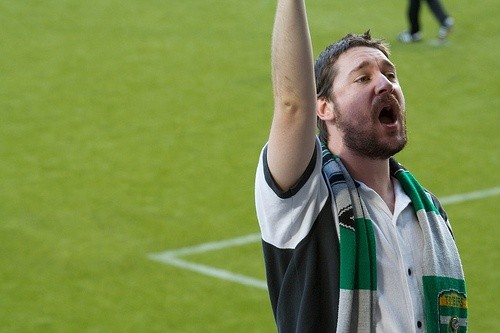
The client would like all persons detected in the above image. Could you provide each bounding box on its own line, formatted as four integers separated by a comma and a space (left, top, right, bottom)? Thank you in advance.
398, 0, 457, 43
255, 1, 468, 333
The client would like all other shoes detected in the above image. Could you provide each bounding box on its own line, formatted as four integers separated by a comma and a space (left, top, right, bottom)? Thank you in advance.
437, 16, 454, 39
398, 32, 421, 42
